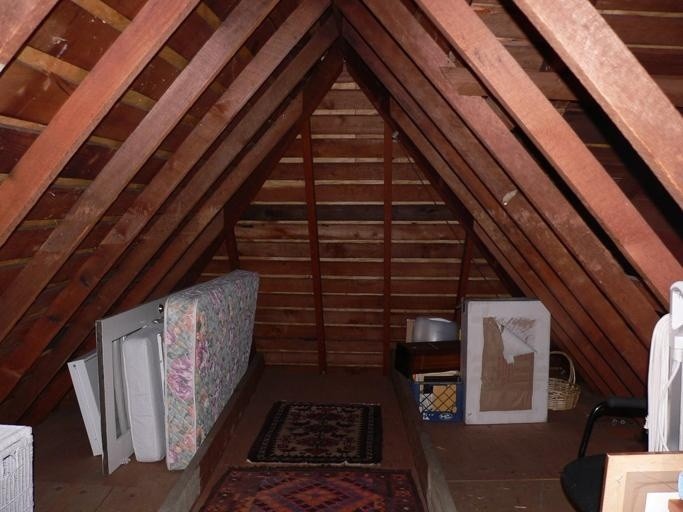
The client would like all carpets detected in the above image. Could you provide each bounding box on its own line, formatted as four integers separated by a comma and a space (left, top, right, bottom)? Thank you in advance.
196, 399, 428, 511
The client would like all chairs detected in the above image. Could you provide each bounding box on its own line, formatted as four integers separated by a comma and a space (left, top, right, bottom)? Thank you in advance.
562, 397, 647, 511
